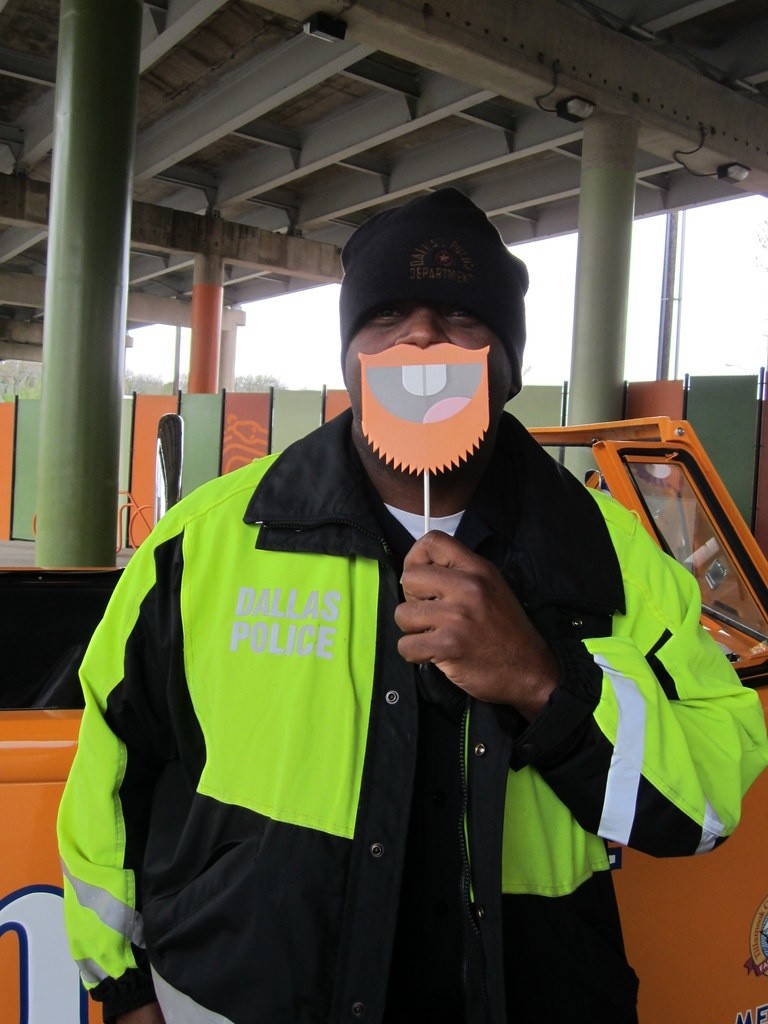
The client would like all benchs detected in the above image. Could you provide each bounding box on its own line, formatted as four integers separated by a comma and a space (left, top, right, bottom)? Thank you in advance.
0, 567, 125, 710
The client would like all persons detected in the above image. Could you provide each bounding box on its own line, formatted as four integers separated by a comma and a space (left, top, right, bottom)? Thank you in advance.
58, 186, 768, 1023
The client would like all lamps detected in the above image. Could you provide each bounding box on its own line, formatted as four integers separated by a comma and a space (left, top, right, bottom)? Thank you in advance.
717, 162, 752, 184
300, 10, 349, 43
556, 94, 597, 124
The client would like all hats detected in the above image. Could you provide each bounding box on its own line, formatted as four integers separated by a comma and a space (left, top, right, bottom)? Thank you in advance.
339, 186, 529, 392
